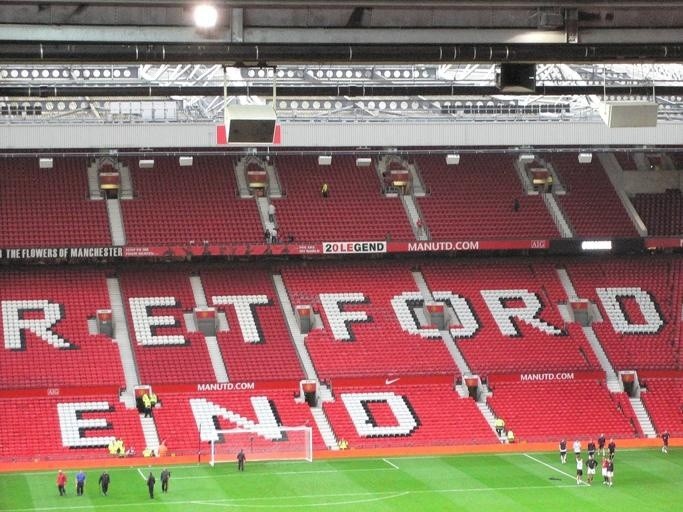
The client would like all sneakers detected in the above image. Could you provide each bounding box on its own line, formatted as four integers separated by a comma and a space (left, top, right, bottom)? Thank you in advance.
588, 479, 594, 487
561, 460, 567, 464
597, 452, 606, 457
602, 481, 613, 488
577, 479, 582, 485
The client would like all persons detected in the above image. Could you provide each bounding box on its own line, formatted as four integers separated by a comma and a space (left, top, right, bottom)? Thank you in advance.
76, 470, 85, 496
383, 230, 392, 242
504, 426, 514, 443
546, 174, 553, 192
149, 391, 158, 406
415, 218, 423, 236
107, 438, 156, 458
511, 193, 520, 212
160, 467, 170, 492
271, 228, 277, 244
558, 433, 616, 488
321, 182, 328, 196
98, 471, 110, 496
660, 430, 670, 454
56, 470, 66, 496
144, 398, 151, 418
268, 202, 275, 222
265, 228, 270, 243
147, 472, 155, 499
337, 437, 348, 450
141, 393, 148, 412
236, 449, 245, 471
493, 416, 504, 437
287, 232, 293, 242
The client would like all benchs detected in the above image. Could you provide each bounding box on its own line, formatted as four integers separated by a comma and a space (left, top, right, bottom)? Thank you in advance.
0, 150, 683, 464
